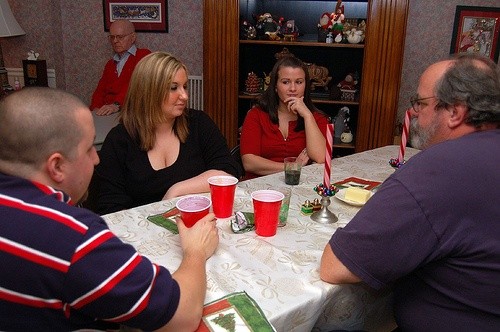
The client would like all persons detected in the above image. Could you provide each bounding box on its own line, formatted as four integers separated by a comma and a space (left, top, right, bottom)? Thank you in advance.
0, 86, 219, 332
317, 12, 333, 31
336, 73, 359, 90
82, 50, 242, 215
319, 51, 500, 332
237, 56, 330, 180
89, 18, 152, 117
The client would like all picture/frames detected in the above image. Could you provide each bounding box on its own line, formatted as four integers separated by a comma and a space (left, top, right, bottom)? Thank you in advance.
102, 0, 169, 34
449, 5, 500, 66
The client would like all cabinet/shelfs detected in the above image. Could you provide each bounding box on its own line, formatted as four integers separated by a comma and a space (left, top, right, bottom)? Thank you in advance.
203, 0, 410, 180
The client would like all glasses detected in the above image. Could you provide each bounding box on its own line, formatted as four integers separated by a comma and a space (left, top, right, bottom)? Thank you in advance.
107, 32, 132, 41
410, 96, 441, 113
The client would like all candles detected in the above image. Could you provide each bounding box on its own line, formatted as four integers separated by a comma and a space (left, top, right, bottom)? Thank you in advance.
393, 107, 411, 167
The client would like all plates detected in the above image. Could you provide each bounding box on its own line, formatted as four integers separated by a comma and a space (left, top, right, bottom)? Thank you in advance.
334, 188, 375, 206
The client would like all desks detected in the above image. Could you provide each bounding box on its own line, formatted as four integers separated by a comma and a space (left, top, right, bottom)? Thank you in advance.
100, 145, 420, 332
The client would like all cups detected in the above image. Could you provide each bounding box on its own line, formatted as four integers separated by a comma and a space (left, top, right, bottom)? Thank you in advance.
266, 186, 291, 227
284, 157, 303, 185
251, 190, 285, 237
208, 175, 239, 219
175, 195, 212, 228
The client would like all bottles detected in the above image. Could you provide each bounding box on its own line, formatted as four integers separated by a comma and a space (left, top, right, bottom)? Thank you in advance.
14, 76, 19, 91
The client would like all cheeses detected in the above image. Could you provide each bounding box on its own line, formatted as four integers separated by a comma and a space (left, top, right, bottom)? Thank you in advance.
345, 186, 370, 203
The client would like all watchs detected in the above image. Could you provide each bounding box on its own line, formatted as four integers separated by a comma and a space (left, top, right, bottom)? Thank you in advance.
113, 102, 121, 111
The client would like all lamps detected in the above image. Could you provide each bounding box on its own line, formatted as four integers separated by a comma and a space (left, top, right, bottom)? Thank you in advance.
0, 0, 27, 89
